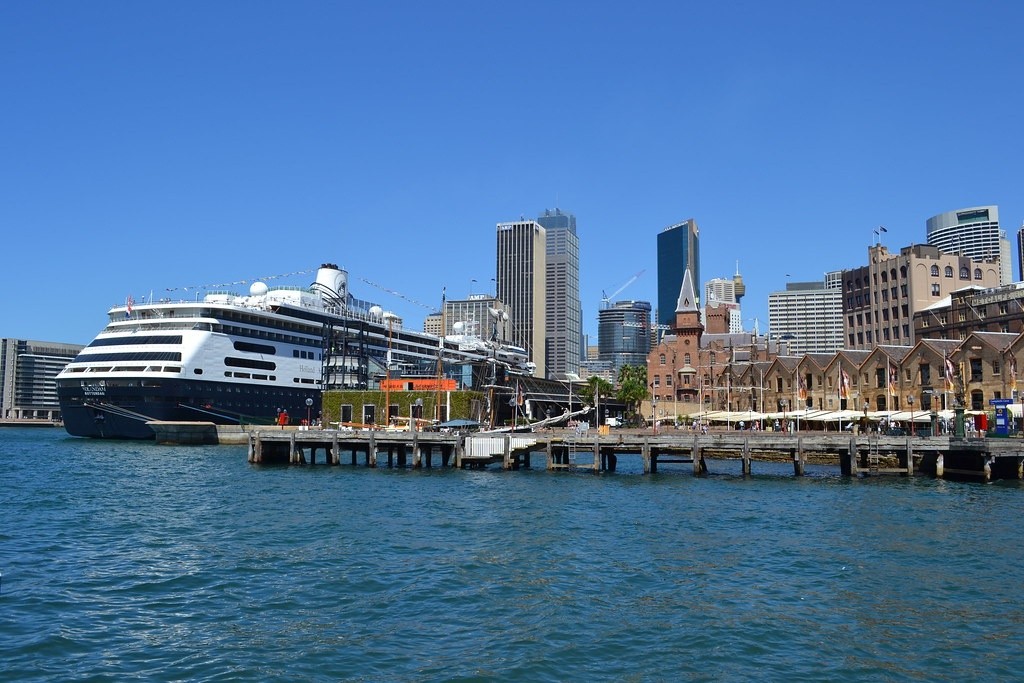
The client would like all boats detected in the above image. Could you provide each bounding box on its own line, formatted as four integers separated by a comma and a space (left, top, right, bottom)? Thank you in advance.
54, 263, 537, 440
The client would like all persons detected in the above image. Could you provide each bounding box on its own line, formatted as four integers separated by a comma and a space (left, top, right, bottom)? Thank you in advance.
880, 418, 885, 429
568, 419, 584, 430
751, 421, 761, 431
675, 421, 679, 428
692, 419, 697, 429
775, 419, 779, 431
890, 419, 901, 430
739, 420, 745, 430
702, 424, 709, 434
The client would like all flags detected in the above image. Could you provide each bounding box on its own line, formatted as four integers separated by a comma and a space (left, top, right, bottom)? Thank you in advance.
840, 368, 850, 399
874, 230, 879, 235
798, 373, 807, 399
889, 366, 896, 396
126, 299, 131, 317
880, 227, 887, 232
1010, 350, 1017, 390
944, 359, 955, 390
518, 384, 523, 406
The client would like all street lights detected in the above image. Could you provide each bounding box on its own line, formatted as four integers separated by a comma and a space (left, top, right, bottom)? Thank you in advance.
951, 398, 957, 430
508, 399, 516, 434
704, 409, 708, 425
385, 314, 397, 426
748, 407, 752, 432
415, 398, 423, 432
546, 409, 551, 430
932, 389, 940, 437
908, 395, 915, 438
650, 400, 657, 436
780, 398, 788, 435
804, 406, 809, 432
665, 409, 669, 430
863, 402, 869, 429
306, 398, 313, 430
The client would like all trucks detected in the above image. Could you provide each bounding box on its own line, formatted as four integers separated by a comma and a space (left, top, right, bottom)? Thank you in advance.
604, 417, 624, 427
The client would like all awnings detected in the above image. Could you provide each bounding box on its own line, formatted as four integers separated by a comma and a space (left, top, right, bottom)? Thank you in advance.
643, 410, 989, 424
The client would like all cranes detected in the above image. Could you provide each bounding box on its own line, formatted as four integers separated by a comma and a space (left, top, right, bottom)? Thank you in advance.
601, 269, 645, 309
